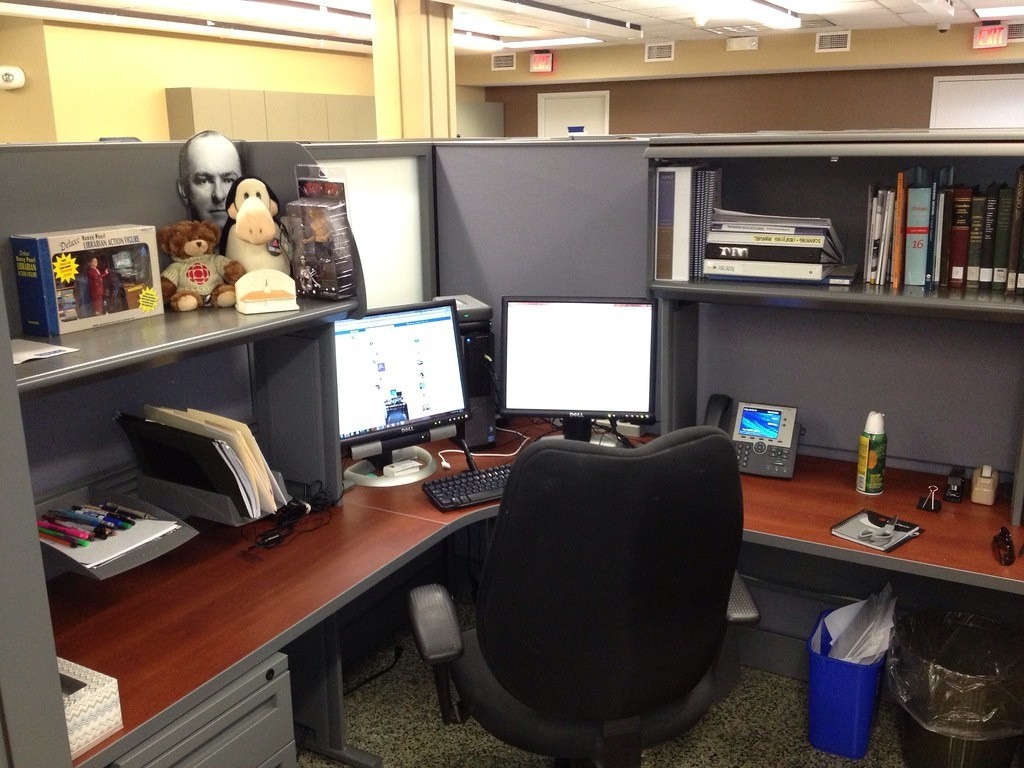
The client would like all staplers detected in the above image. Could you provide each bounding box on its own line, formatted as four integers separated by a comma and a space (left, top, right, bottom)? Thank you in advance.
943, 464, 967, 503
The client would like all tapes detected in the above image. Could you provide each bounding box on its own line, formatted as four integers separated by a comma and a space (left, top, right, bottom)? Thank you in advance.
982, 465, 993, 478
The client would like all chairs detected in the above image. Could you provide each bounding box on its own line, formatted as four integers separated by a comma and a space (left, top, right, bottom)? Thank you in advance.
408, 429, 759, 768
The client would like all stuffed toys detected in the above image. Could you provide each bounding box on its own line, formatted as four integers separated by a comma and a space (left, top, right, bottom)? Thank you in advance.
157, 175, 292, 313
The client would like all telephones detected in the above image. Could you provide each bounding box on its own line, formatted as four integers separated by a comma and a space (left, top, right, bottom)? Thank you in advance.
703, 392, 807, 480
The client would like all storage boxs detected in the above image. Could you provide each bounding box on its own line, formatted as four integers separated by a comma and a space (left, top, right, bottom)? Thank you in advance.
8, 222, 165, 340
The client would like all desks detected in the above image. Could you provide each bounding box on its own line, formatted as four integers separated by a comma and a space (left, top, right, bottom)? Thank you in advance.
341, 417, 1024, 599
40, 491, 446, 768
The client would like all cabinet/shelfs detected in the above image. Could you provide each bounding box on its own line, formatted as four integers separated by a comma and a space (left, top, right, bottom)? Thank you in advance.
108, 651, 298, 768
342, 143, 1024, 679
0, 138, 448, 768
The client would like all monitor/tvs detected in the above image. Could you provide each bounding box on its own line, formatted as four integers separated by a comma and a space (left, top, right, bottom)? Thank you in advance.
334, 298, 471, 488
499, 297, 659, 450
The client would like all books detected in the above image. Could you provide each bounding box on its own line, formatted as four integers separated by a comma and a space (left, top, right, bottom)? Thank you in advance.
830, 508, 920, 551
703, 164, 1024, 300
39, 508, 178, 568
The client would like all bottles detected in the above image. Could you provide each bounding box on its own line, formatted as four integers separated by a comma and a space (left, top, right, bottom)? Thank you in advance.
855, 410, 888, 495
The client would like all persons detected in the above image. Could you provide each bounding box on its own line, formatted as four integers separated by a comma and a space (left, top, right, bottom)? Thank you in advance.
88, 257, 109, 315
302, 208, 339, 291
177, 130, 243, 235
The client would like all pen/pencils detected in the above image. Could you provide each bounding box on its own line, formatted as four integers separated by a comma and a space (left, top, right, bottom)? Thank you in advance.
37, 502, 159, 549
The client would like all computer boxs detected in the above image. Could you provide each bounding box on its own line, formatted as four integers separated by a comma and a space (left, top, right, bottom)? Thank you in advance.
448, 330, 496, 451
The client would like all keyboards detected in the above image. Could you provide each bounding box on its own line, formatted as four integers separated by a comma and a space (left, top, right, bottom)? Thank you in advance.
422, 464, 514, 513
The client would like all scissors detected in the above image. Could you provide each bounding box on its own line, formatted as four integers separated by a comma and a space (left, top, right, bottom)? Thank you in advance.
857, 513, 900, 542
